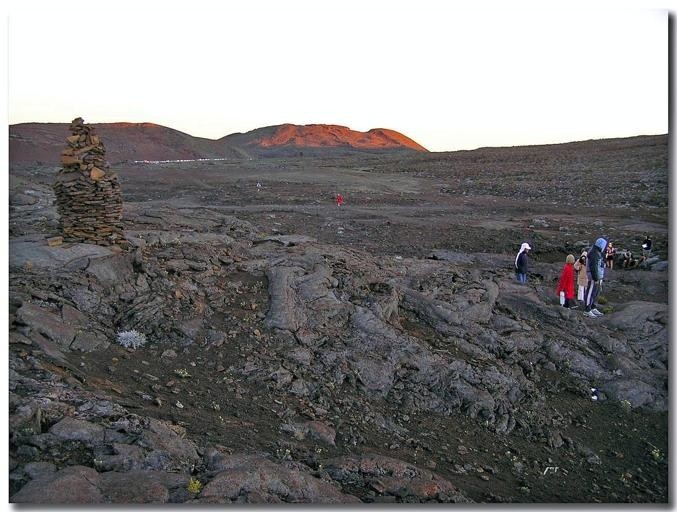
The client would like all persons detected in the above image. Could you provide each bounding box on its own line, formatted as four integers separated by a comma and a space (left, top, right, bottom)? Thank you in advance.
515, 242, 531, 283
556, 254, 575, 307
573, 236, 653, 318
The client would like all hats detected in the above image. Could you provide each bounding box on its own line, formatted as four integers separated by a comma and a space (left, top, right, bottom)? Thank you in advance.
521, 243, 531, 250
608, 243, 612, 248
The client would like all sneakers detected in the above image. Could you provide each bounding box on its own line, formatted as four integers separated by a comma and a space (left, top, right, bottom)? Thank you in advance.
581, 308, 604, 319
